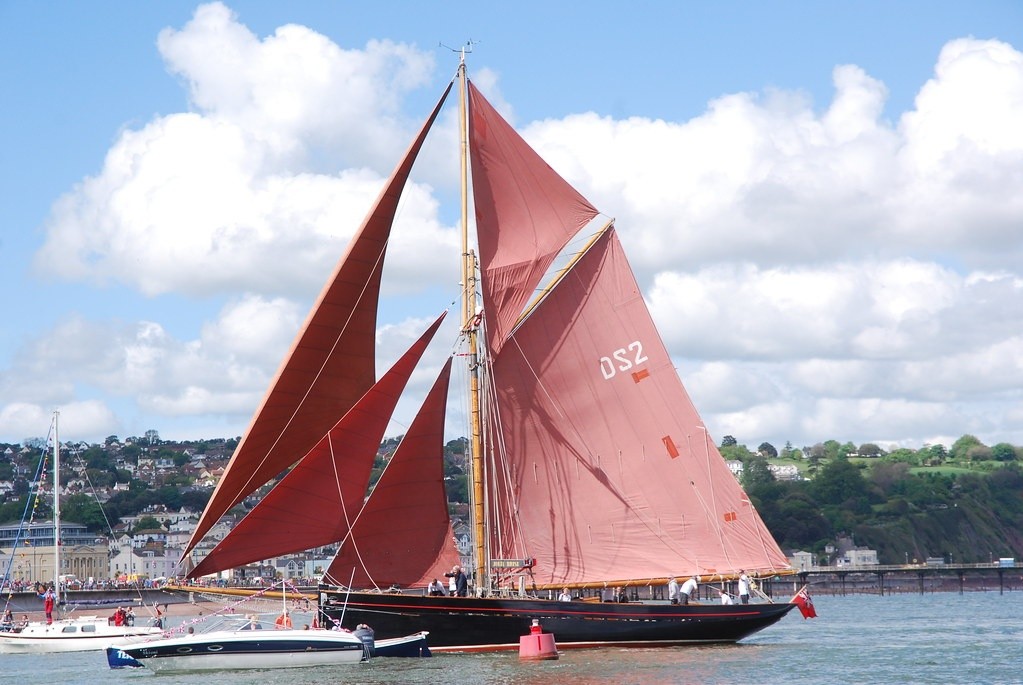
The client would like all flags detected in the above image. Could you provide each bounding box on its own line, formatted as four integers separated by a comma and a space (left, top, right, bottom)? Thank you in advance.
793, 586, 819, 620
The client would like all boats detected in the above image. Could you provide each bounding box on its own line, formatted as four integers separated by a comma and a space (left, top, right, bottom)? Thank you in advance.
106, 578, 373, 673
354, 630, 434, 660
60, 598, 145, 611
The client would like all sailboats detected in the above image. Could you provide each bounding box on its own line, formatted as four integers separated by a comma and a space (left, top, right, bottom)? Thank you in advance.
0, 410, 166, 655
175, 43, 807, 658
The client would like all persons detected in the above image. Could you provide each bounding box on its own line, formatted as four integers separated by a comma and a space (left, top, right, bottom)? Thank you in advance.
719, 589, 733, 605
679, 576, 698, 605
738, 569, 749, 604
37, 587, 57, 625
558, 588, 571, 602
443, 565, 467, 597
0, 609, 30, 633
241, 614, 262, 630
184, 627, 195, 638
5, 575, 321, 593
667, 574, 680, 605
271, 609, 291, 630
108, 606, 137, 626
428, 578, 446, 597
613, 586, 629, 603
